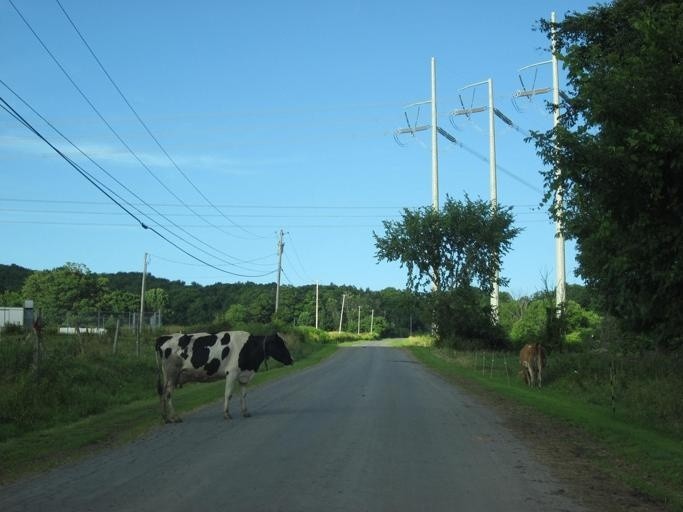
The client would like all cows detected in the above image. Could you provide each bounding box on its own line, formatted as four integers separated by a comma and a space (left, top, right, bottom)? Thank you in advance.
154, 330, 294, 423
519, 342, 549, 390
